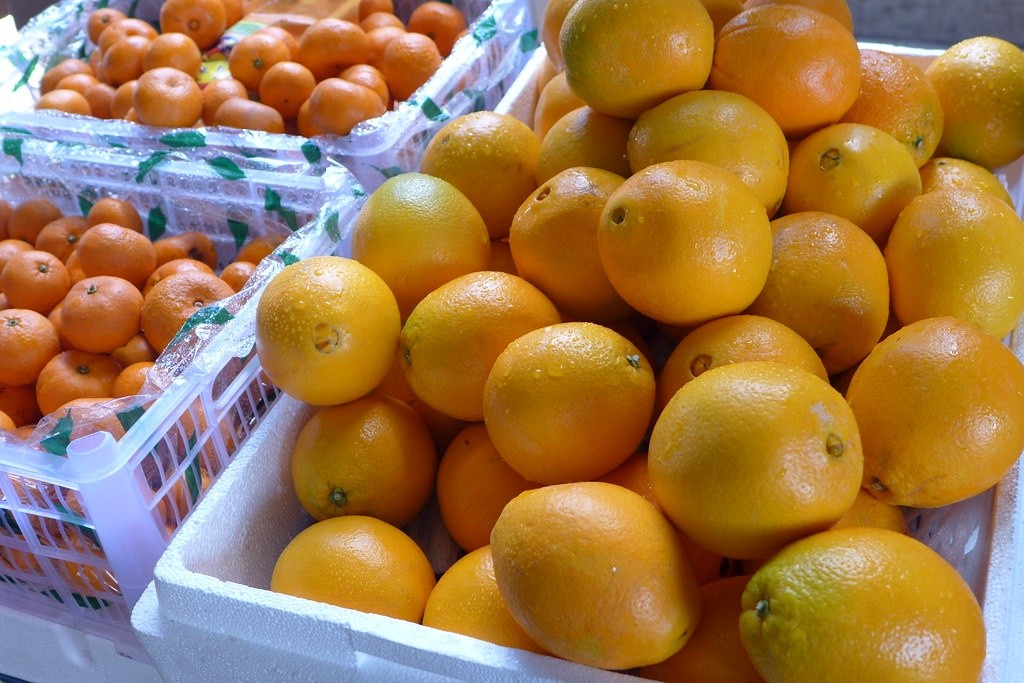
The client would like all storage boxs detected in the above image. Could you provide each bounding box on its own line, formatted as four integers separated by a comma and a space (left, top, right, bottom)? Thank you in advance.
130, 37, 1022, 679
0, 152, 370, 646
0, 1, 542, 187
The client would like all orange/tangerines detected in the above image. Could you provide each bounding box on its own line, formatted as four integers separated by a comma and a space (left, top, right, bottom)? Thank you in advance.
1, 198, 285, 595
254, 2, 1024, 683
31, 0, 473, 135
